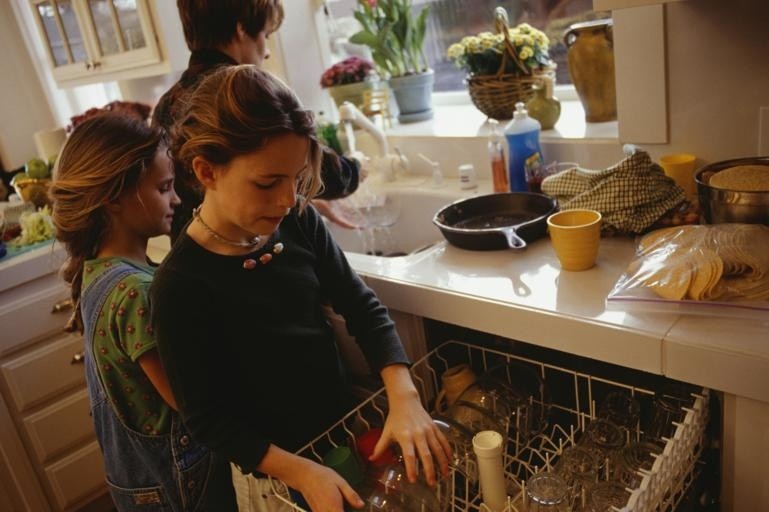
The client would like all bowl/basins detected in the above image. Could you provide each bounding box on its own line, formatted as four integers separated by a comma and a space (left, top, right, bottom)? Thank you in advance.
696, 154, 769, 226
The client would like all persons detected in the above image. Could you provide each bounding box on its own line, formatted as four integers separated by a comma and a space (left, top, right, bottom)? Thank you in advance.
148, 62, 455, 512
150, 0, 372, 250
44, 110, 237, 512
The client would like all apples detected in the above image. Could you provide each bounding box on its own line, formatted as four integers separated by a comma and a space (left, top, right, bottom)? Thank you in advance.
11, 155, 55, 187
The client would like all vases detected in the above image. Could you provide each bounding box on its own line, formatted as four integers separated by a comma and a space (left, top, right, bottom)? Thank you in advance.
524, 82, 561, 131
387, 67, 435, 124
327, 80, 372, 131
562, 18, 618, 125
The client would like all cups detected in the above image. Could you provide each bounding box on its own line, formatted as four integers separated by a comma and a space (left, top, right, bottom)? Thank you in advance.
546, 208, 602, 272
658, 154, 696, 179
321, 362, 681, 511
458, 164, 476, 188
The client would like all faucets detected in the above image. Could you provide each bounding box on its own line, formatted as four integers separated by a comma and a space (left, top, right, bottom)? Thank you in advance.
340, 102, 408, 183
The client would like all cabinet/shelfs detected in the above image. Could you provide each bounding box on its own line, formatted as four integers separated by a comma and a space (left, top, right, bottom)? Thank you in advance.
0, 227, 114, 512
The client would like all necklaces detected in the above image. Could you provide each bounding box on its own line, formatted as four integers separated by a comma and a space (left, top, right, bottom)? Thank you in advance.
190, 202, 264, 248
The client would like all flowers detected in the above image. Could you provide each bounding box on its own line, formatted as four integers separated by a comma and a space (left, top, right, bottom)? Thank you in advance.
320, 55, 376, 89
349, 0, 433, 78
444, 21, 551, 72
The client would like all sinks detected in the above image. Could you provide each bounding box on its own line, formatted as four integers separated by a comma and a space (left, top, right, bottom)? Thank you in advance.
319, 188, 465, 258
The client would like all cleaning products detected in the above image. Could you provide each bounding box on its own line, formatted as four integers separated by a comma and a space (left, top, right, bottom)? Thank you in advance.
504, 102, 543, 193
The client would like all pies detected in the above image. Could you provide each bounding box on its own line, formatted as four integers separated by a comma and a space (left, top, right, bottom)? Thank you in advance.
710, 166, 769, 192
626, 224, 769, 302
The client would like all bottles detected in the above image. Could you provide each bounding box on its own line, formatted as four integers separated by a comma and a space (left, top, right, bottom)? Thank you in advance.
314, 108, 340, 154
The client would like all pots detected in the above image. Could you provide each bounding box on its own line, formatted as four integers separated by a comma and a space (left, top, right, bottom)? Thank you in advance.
432, 190, 558, 252
